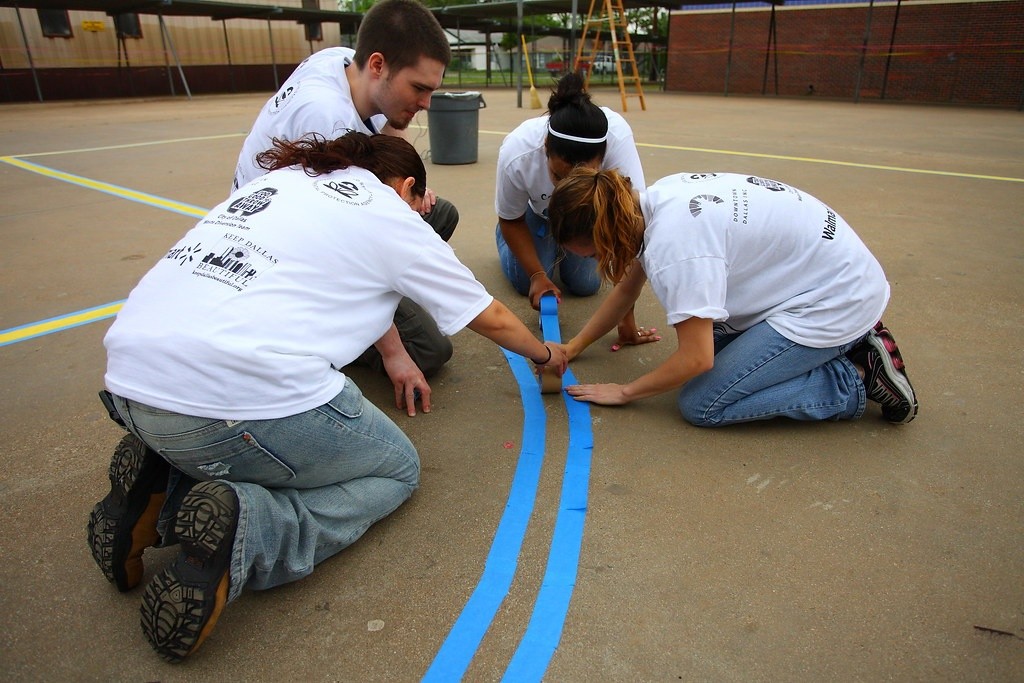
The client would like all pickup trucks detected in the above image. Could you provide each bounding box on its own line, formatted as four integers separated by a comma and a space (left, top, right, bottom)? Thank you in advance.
590, 55, 617, 74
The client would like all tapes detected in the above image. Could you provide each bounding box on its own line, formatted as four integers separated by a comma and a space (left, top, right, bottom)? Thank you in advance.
537, 296, 561, 331
537, 364, 565, 397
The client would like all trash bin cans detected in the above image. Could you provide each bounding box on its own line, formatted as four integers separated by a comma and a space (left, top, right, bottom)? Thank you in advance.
427, 90, 487, 166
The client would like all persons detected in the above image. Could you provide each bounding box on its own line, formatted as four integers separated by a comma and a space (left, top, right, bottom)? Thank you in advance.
542, 163, 918, 429
495, 72, 662, 352
229, 0, 460, 416
83, 131, 570, 666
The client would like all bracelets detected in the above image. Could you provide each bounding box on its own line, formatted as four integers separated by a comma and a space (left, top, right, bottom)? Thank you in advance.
531, 345, 552, 365
530, 271, 546, 281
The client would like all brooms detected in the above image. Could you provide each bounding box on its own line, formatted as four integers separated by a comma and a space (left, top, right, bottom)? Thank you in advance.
521, 34, 544, 110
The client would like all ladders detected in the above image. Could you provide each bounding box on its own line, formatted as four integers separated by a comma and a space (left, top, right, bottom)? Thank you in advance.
569, 0, 647, 114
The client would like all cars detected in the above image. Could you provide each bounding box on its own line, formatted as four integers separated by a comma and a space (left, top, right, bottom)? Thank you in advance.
546, 56, 589, 78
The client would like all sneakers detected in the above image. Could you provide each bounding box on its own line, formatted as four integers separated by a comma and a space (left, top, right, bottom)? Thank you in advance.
87, 432, 171, 593
847, 321, 919, 426
139, 480, 240, 666
